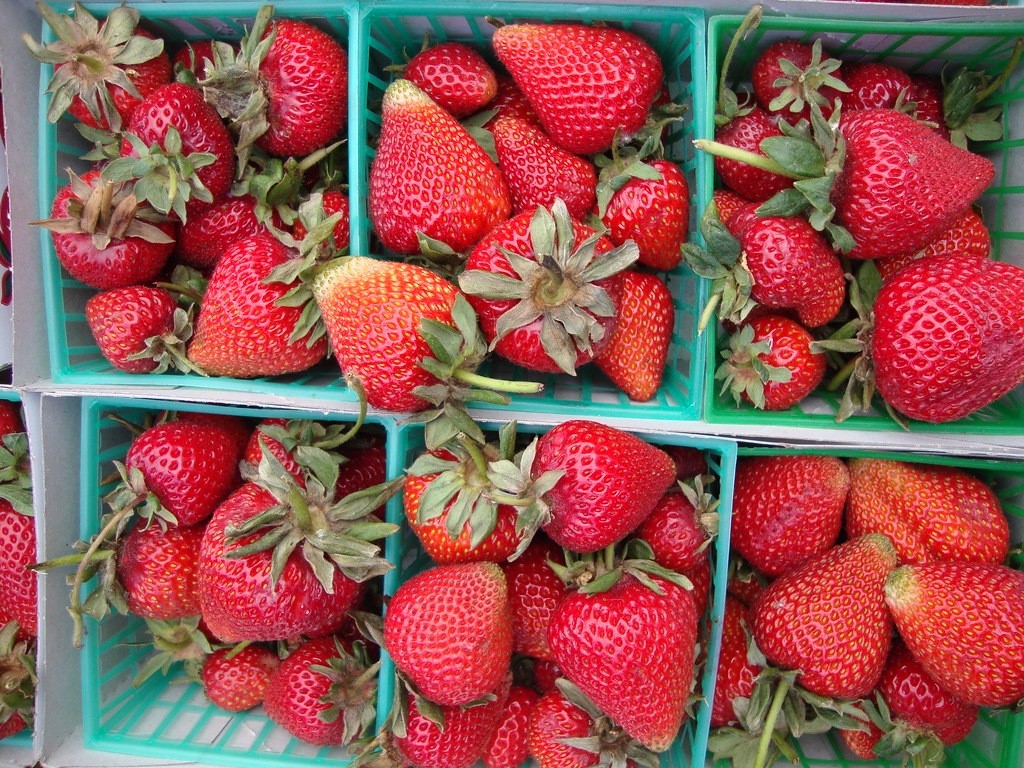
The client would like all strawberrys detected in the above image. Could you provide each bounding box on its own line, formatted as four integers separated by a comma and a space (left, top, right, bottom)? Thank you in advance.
0, 1, 1024, 768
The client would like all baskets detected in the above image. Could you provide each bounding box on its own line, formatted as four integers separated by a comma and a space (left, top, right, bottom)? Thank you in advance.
0, 0, 1024, 768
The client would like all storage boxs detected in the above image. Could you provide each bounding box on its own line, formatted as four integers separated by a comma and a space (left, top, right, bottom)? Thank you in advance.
0, 0, 1023, 768
